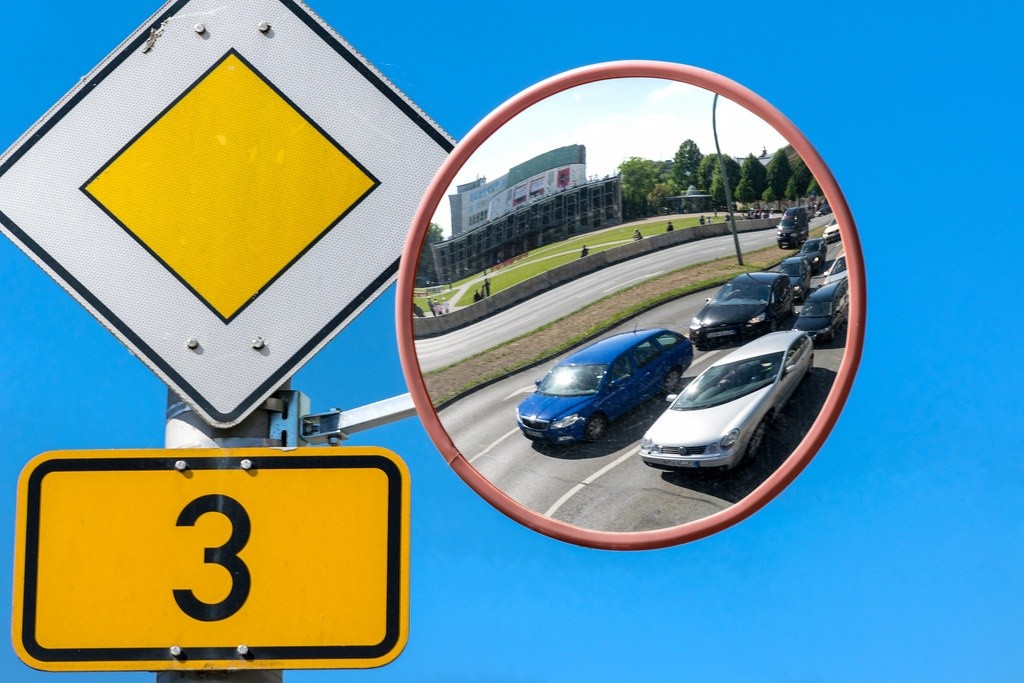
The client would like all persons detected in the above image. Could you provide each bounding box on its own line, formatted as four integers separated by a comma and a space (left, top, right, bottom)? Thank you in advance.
580, 245, 590, 258
413, 278, 490, 317
632, 228, 643, 241
699, 190, 829, 258
667, 221, 674, 232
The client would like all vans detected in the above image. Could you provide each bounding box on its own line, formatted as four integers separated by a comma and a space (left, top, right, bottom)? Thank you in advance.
775, 206, 809, 249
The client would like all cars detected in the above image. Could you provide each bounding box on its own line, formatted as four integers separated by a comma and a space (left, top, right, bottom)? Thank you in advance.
638, 330, 815, 472
514, 326, 695, 446
814, 203, 833, 217
688, 270, 796, 352
816, 248, 848, 287
795, 237, 829, 266
822, 216, 842, 244
790, 275, 850, 347
778, 251, 828, 304
